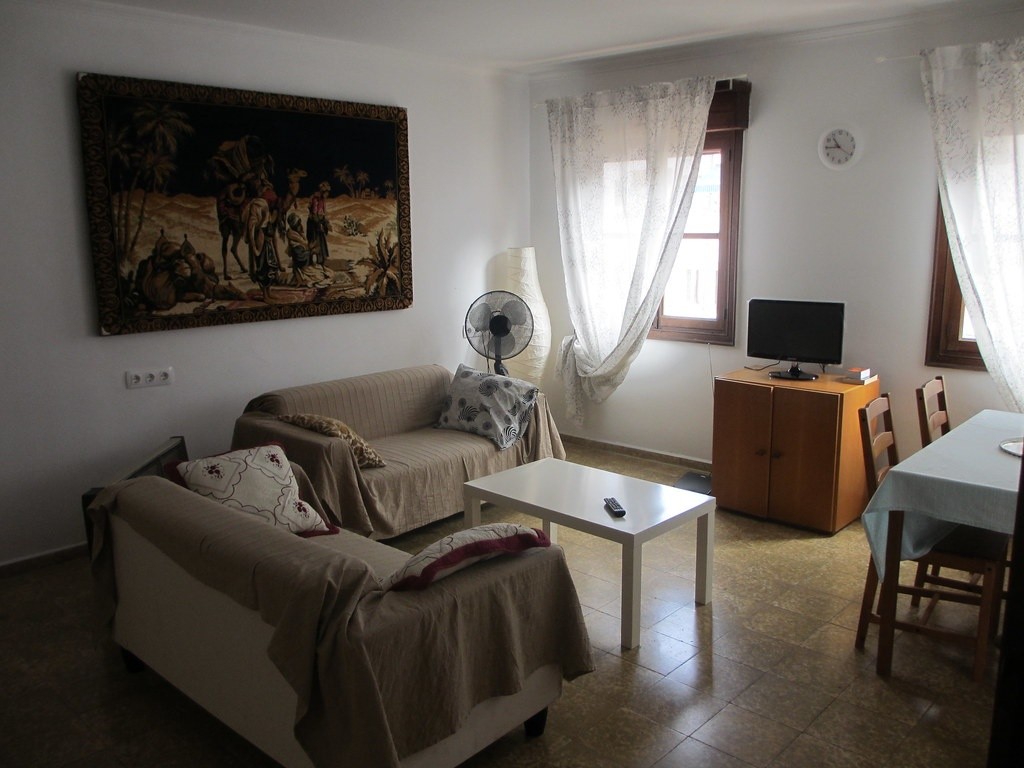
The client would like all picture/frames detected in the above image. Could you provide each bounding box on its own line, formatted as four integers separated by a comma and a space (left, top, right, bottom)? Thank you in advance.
74, 72, 414, 336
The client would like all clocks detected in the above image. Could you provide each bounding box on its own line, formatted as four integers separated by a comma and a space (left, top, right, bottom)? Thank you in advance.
818, 124, 863, 171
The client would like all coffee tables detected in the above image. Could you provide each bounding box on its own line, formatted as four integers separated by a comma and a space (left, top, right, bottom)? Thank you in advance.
464, 457, 716, 649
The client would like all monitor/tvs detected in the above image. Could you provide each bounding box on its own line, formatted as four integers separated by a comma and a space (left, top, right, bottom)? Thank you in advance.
746, 299, 845, 381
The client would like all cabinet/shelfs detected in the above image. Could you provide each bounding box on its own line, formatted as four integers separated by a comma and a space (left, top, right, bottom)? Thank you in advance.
712, 364, 881, 536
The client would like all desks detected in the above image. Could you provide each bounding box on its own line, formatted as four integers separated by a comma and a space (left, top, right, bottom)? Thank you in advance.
862, 409, 1024, 676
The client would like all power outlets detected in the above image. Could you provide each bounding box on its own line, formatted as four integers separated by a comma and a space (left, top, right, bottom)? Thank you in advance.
463, 325, 489, 338
125, 365, 173, 390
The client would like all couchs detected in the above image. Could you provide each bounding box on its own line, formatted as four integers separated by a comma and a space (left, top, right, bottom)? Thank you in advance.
86, 460, 597, 768
231, 363, 566, 541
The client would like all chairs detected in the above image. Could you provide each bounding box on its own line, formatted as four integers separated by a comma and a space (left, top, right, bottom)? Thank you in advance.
910, 375, 1013, 608
854, 392, 1007, 683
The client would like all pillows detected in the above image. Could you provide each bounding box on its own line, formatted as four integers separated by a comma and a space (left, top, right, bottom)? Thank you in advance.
163, 442, 341, 539
432, 364, 539, 451
377, 523, 551, 592
276, 413, 386, 469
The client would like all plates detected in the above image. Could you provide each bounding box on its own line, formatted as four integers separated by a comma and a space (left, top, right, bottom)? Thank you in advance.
1000, 437, 1024, 457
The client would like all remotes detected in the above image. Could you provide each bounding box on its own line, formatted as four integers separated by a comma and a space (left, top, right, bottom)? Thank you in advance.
604, 497, 626, 517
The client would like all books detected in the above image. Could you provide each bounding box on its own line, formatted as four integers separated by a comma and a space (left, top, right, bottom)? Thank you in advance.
841, 368, 878, 385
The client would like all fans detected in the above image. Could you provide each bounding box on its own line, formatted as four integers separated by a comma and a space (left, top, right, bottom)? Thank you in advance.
464, 290, 534, 375
